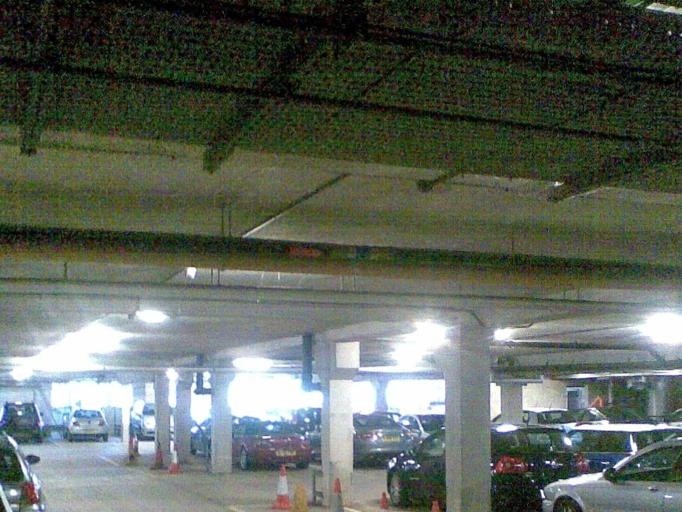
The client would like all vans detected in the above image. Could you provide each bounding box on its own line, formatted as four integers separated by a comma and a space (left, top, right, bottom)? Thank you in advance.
132, 400, 176, 441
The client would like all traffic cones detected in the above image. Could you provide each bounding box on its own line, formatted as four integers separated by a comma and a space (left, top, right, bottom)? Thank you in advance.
150, 442, 182, 473
272, 466, 291, 508
329, 479, 344, 512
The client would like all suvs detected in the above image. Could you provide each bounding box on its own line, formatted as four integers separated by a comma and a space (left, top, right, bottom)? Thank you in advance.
2, 402, 44, 444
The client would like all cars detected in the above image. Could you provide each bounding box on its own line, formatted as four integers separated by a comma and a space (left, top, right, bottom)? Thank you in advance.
65, 409, 110, 441
189, 408, 322, 469
0, 435, 46, 512
